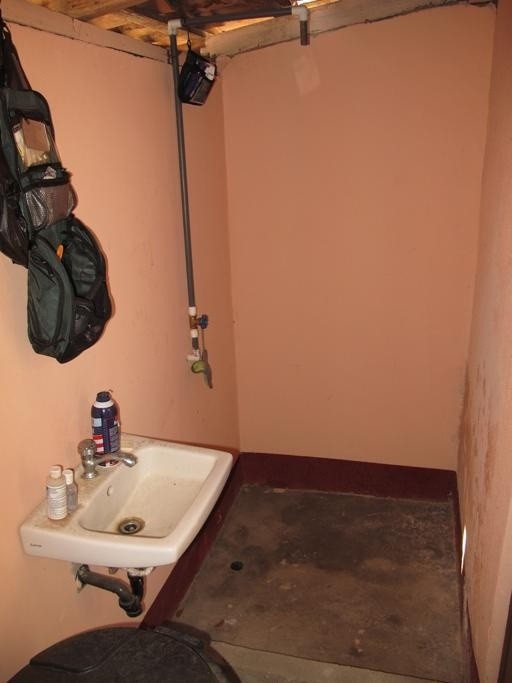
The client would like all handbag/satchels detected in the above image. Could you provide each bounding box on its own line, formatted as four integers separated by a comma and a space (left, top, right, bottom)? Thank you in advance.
0, 84, 113, 365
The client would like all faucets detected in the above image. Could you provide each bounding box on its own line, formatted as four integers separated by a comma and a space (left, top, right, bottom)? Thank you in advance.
78, 440, 137, 479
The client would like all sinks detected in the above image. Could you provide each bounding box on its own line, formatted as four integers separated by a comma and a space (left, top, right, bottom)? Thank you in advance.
19, 432, 233, 569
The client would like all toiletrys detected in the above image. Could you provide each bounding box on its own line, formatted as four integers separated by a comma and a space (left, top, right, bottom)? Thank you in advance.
45, 465, 68, 520
64, 469, 79, 509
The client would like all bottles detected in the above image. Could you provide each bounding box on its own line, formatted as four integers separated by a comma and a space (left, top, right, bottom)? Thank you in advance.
91, 391, 120, 468
45, 465, 78, 522
